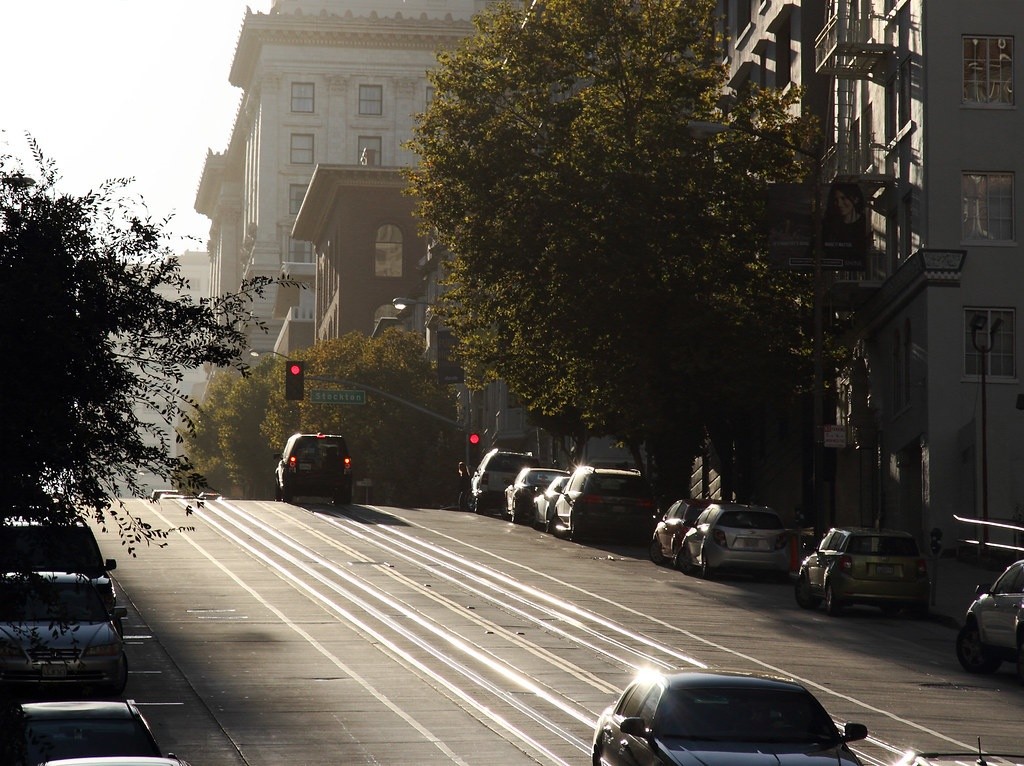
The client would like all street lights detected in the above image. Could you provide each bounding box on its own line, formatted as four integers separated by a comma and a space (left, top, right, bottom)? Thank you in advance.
969, 313, 1005, 560
684, 118, 824, 545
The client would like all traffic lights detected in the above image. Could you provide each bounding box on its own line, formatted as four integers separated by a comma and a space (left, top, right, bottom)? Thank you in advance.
470, 434, 479, 458
286, 361, 304, 401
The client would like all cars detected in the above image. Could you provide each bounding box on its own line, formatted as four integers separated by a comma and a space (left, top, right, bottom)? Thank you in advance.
673, 503, 792, 581
648, 498, 722, 566
532, 476, 570, 534
150, 489, 230, 500
0, 518, 192, 766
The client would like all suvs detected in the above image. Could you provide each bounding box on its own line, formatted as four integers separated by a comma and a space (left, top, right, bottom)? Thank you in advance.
272, 432, 354, 505
465, 448, 541, 515
955, 559, 1024, 673
501, 467, 568, 522
554, 464, 651, 542
794, 526, 931, 620
591, 668, 868, 766
